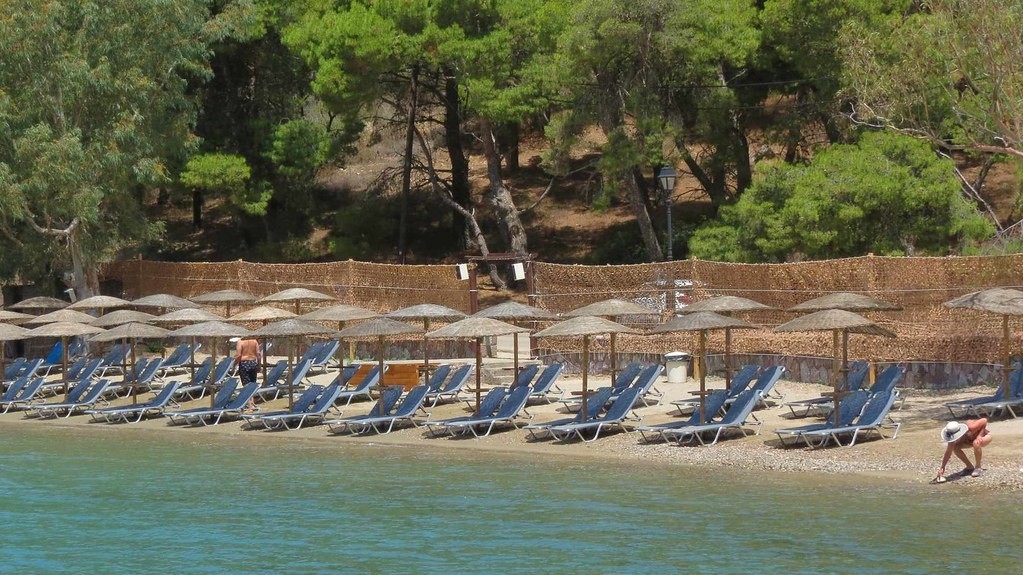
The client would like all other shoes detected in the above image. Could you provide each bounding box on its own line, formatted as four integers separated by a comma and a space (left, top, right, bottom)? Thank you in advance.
972, 469, 983, 476
959, 468, 974, 476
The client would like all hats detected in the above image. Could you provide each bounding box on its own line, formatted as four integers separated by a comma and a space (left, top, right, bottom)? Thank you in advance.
941, 421, 968, 442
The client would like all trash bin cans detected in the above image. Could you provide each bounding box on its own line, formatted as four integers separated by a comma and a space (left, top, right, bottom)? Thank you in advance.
663, 350, 690, 383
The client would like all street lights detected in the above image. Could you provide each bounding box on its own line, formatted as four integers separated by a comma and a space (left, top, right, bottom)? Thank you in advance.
659, 161, 677, 310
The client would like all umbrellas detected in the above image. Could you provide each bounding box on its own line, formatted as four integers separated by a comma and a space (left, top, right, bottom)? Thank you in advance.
227, 302, 300, 399
559, 298, 664, 388
90, 310, 158, 385
253, 287, 337, 363
86, 319, 177, 417
166, 319, 255, 421
470, 299, 559, 382
147, 307, 227, 399
644, 311, 763, 440
187, 288, 262, 358
23, 307, 97, 381
672, 295, 781, 408
3, 295, 73, 360
0, 322, 32, 413
240, 316, 339, 426
786, 292, 904, 391
380, 303, 468, 405
0, 310, 38, 326
65, 296, 132, 328
329, 317, 426, 430
942, 287, 1023, 400
773, 308, 897, 438
21, 321, 109, 415
529, 314, 644, 437
294, 304, 384, 386
128, 293, 201, 377
424, 315, 532, 433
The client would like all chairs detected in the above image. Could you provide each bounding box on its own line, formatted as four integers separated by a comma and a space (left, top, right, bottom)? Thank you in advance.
556, 364, 665, 417
638, 389, 765, 447
784, 361, 907, 418
944, 362, 1023, 420
773, 390, 901, 449
523, 386, 642, 444
1, 338, 562, 440
671, 365, 787, 418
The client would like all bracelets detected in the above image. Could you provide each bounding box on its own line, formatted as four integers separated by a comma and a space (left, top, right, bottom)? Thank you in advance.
941, 466, 945, 469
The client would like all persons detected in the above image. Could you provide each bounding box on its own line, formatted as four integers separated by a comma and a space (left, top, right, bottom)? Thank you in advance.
936, 417, 994, 481
233, 339, 261, 414
